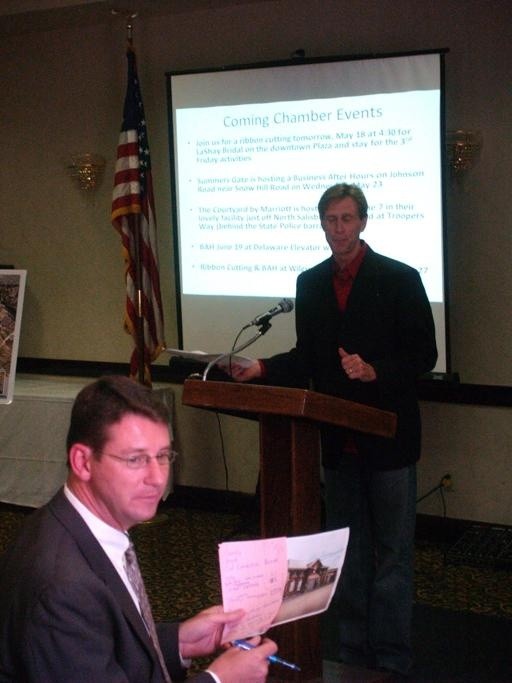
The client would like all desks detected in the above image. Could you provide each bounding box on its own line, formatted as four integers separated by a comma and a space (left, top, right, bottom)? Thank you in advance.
0, 368, 177, 515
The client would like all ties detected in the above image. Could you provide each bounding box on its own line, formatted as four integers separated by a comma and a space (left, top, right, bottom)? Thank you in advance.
124, 547, 173, 681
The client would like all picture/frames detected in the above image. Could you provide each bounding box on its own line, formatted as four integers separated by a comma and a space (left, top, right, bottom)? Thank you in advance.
1, 268, 28, 405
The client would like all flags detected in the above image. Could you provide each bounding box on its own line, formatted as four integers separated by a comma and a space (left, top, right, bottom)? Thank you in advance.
112, 42, 167, 388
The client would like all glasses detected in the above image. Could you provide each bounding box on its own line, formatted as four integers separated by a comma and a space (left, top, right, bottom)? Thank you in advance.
94, 449, 178, 470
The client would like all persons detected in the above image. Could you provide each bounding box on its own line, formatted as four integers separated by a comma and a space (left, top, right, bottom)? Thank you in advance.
219, 184, 438, 683
0, 375, 279, 683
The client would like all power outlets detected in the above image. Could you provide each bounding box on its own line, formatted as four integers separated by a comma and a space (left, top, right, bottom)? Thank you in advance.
439, 466, 456, 495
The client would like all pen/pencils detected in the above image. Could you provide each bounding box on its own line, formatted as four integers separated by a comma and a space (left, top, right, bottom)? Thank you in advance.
232, 641, 302, 671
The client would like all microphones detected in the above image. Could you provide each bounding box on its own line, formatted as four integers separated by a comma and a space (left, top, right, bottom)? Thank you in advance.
246, 297, 294, 326
